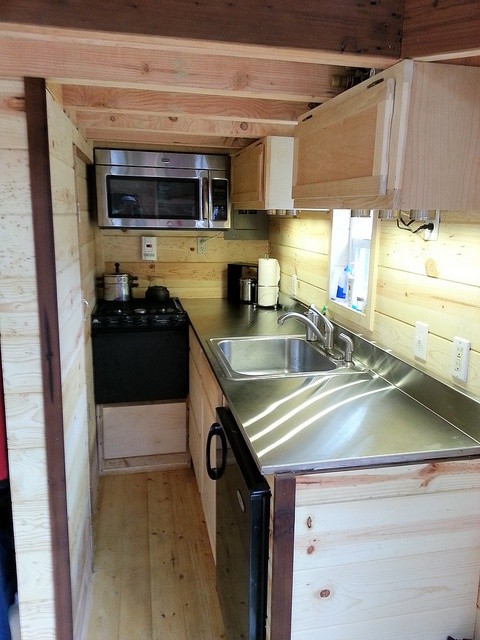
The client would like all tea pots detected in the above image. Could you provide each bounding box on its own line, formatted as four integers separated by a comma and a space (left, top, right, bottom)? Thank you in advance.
144, 275, 171, 309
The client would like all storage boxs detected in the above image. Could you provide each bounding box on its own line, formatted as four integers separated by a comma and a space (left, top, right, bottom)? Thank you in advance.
231, 133, 294, 210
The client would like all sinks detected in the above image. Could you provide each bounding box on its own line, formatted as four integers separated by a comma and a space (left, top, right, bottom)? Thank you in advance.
213, 335, 340, 378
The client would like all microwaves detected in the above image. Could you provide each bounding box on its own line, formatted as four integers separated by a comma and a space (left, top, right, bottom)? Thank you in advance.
91, 149, 232, 230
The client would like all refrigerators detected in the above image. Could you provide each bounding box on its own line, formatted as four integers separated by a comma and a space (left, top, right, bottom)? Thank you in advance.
204, 404, 273, 547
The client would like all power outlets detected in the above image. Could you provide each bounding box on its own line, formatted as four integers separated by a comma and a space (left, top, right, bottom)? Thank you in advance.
413, 322, 428, 362
197, 236, 207, 255
451, 335, 470, 383
423, 220, 440, 241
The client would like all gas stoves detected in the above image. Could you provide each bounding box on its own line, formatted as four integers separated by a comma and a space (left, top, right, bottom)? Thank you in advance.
94, 297, 186, 322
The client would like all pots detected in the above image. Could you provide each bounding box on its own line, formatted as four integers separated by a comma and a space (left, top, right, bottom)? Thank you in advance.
96, 263, 139, 303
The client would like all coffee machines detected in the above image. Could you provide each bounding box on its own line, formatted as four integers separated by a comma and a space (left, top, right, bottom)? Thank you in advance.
225, 262, 259, 303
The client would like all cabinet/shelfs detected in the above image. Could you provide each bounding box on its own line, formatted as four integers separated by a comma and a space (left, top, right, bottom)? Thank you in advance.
188, 325, 235, 564
291, 59, 480, 213
95, 402, 189, 476
